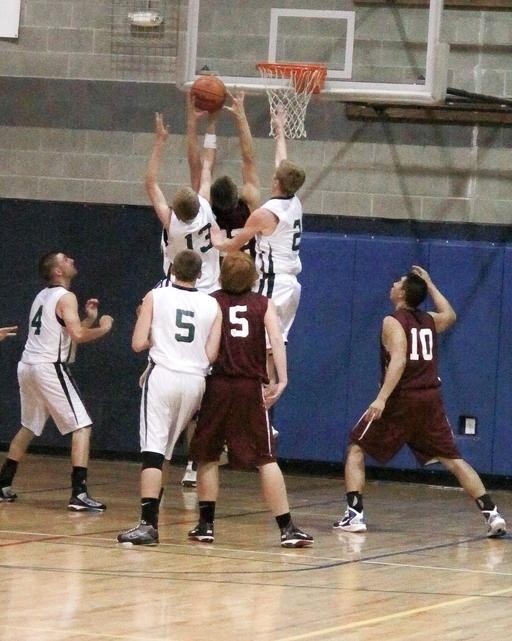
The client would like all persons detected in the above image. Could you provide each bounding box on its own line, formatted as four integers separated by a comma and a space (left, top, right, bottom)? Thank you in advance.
1, 248, 114, 516
330, 265, 508, 541
209, 103, 306, 441
116, 250, 224, 550
187, 252, 316, 549
184, 85, 260, 273
144, 96, 224, 488
0, 323, 22, 345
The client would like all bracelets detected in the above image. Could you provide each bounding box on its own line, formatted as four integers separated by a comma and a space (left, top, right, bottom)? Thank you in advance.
202, 132, 221, 155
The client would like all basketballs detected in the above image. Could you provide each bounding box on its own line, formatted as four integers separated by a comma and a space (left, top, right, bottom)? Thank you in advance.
190, 75, 226, 110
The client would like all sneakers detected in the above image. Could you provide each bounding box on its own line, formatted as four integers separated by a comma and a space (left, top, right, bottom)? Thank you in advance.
117, 524, 159, 546
181, 461, 197, 487
482, 505, 506, 538
67, 492, 106, 512
188, 521, 214, 543
334, 506, 367, 533
0, 487, 17, 502
281, 523, 314, 548
